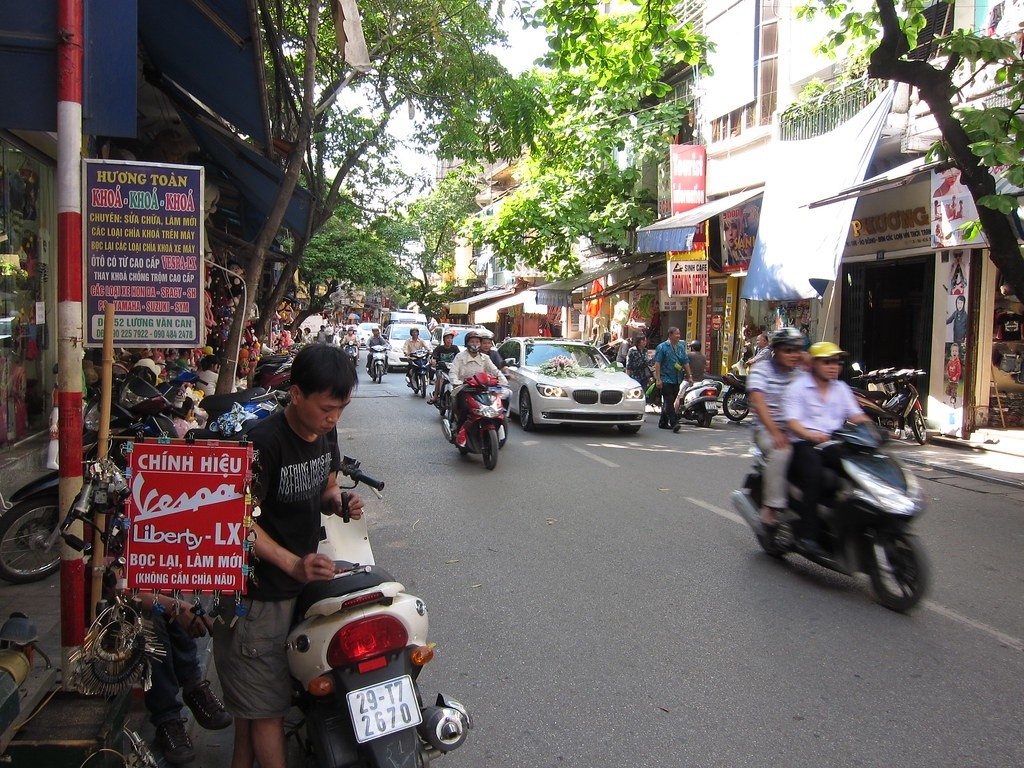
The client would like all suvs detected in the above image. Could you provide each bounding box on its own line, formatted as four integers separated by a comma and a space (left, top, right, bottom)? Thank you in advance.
430, 323, 497, 352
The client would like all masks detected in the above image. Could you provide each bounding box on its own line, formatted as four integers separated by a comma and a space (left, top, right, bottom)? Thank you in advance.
469, 345, 481, 353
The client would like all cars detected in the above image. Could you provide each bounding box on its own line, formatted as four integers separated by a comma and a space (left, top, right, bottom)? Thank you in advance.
358, 323, 380, 343
495, 336, 646, 434
382, 324, 431, 370
333, 324, 358, 348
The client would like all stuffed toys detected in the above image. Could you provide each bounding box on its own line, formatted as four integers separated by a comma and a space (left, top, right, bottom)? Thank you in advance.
144, 181, 295, 377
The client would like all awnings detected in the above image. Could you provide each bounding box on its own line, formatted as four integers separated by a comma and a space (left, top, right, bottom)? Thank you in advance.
448, 265, 639, 325
808, 131, 1023, 209
136, 0, 317, 261
635, 186, 764, 253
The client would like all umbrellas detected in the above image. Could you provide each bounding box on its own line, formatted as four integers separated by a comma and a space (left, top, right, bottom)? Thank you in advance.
344, 313, 360, 325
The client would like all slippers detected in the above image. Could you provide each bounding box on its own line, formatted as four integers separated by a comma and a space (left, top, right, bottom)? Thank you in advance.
426, 398, 438, 405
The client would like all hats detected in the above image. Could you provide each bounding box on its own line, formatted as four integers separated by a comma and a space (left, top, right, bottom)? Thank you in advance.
202, 346, 216, 356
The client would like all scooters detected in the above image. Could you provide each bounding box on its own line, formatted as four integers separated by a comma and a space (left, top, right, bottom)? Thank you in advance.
733, 421, 930, 612
252, 350, 292, 405
681, 378, 721, 428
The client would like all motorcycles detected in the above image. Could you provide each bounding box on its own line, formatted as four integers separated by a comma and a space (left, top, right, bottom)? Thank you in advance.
0, 358, 475, 768
400, 351, 432, 398
344, 338, 364, 366
723, 372, 752, 423
366, 345, 390, 384
441, 361, 511, 470
844, 363, 928, 445
430, 358, 454, 422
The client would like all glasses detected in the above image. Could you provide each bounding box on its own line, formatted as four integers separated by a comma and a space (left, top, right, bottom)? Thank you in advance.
779, 348, 802, 354
822, 358, 845, 366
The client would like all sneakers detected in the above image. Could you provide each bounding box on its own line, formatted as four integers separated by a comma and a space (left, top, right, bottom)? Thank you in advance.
182, 679, 233, 730
155, 716, 196, 764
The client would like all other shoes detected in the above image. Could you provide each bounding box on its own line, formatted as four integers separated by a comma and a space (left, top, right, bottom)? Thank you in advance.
455, 426, 468, 448
757, 504, 779, 527
673, 423, 681, 432
384, 369, 388, 374
659, 424, 672, 429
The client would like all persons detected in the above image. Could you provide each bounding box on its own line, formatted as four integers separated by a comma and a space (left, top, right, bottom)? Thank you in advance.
775, 342, 876, 558
197, 353, 221, 398
585, 326, 770, 434
944, 250, 966, 405
173, 348, 196, 371
933, 168, 963, 246
744, 327, 811, 526
85, 344, 357, 768
294, 322, 517, 433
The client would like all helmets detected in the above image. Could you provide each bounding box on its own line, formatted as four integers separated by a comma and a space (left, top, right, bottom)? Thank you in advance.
464, 330, 481, 346
410, 328, 420, 335
348, 326, 354, 333
810, 342, 849, 358
688, 340, 701, 351
480, 328, 496, 340
443, 330, 458, 338
133, 358, 162, 386
768, 327, 809, 346
372, 328, 379, 333
